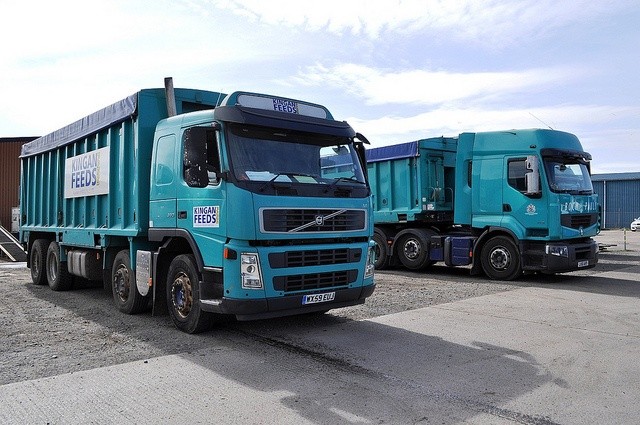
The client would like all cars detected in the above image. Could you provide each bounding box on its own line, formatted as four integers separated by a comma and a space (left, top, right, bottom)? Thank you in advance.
630, 217, 640, 231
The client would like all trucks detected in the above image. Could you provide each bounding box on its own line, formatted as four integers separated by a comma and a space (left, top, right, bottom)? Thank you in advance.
321, 128, 602, 281
19, 77, 377, 333
